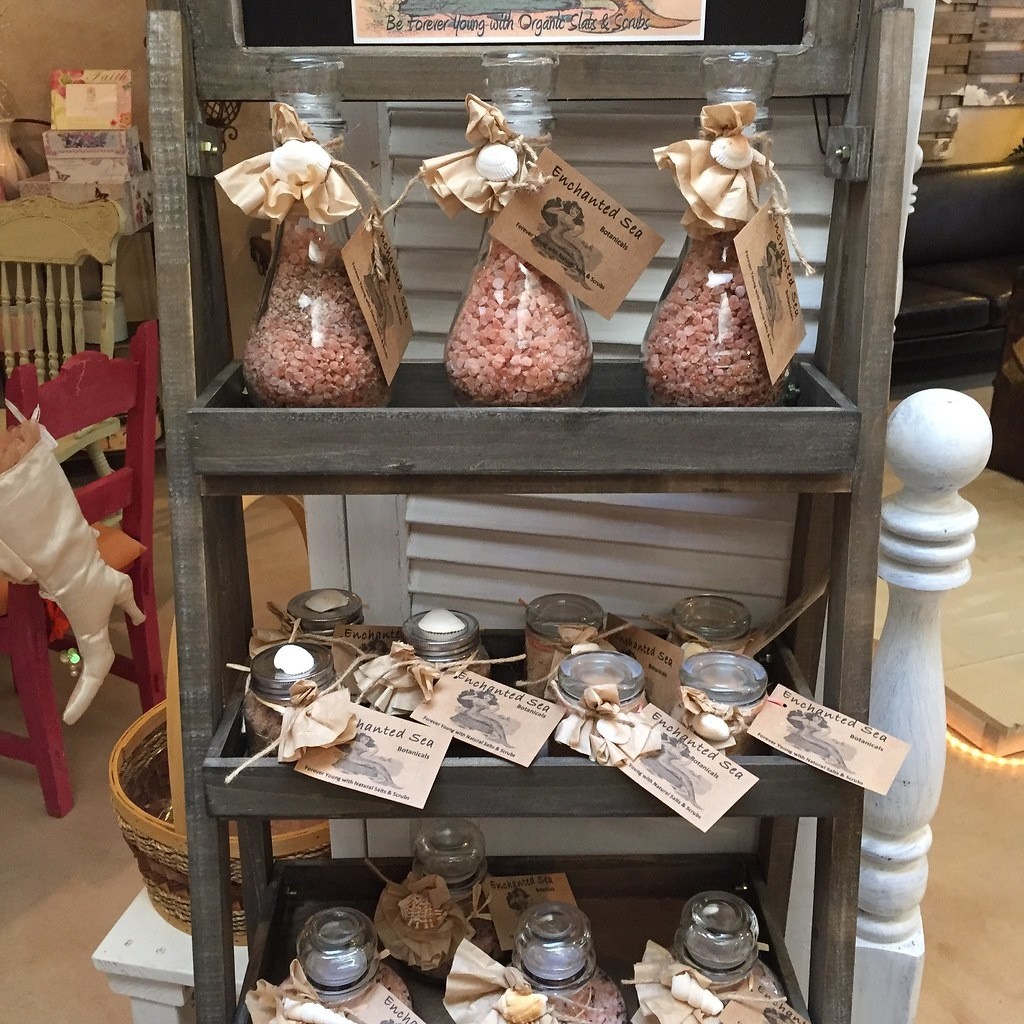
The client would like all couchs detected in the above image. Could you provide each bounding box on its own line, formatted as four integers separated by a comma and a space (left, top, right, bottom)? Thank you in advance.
890, 165, 1023, 382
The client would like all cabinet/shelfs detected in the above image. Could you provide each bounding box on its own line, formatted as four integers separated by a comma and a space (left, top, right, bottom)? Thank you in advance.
92, 884, 252, 1024
2, 232, 158, 322
146, 1, 918, 1024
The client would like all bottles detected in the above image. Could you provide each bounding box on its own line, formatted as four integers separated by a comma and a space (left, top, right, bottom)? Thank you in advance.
669, 649, 769, 755
444, 48, 595, 408
401, 608, 492, 757
506, 900, 627, 1024
286, 586, 364, 669
667, 891, 793, 1024
661, 594, 750, 739
243, 92, 394, 410
245, 639, 339, 757
639, 53, 793, 408
246, 908, 413, 1024
406, 817, 502, 981
551, 650, 647, 759
525, 592, 603, 756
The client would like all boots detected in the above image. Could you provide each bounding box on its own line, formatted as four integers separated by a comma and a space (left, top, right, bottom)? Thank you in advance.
0, 420, 147, 723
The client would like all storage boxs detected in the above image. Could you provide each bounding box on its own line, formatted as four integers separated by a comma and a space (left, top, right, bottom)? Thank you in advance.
43, 127, 142, 182
108, 691, 336, 945
18, 173, 153, 235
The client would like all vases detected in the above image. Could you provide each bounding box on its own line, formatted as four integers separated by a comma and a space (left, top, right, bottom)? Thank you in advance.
0, 120, 32, 200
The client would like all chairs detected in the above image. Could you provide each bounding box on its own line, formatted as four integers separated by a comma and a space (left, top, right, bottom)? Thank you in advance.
0, 197, 122, 481
2, 323, 165, 817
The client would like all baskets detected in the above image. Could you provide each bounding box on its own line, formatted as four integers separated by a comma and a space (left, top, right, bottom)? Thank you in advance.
109, 697, 332, 944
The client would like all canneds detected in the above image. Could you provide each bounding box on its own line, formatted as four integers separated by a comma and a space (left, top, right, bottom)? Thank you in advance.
241, 586, 769, 756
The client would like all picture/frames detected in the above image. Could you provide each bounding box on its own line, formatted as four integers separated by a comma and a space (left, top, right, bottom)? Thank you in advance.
49, 69, 131, 131
